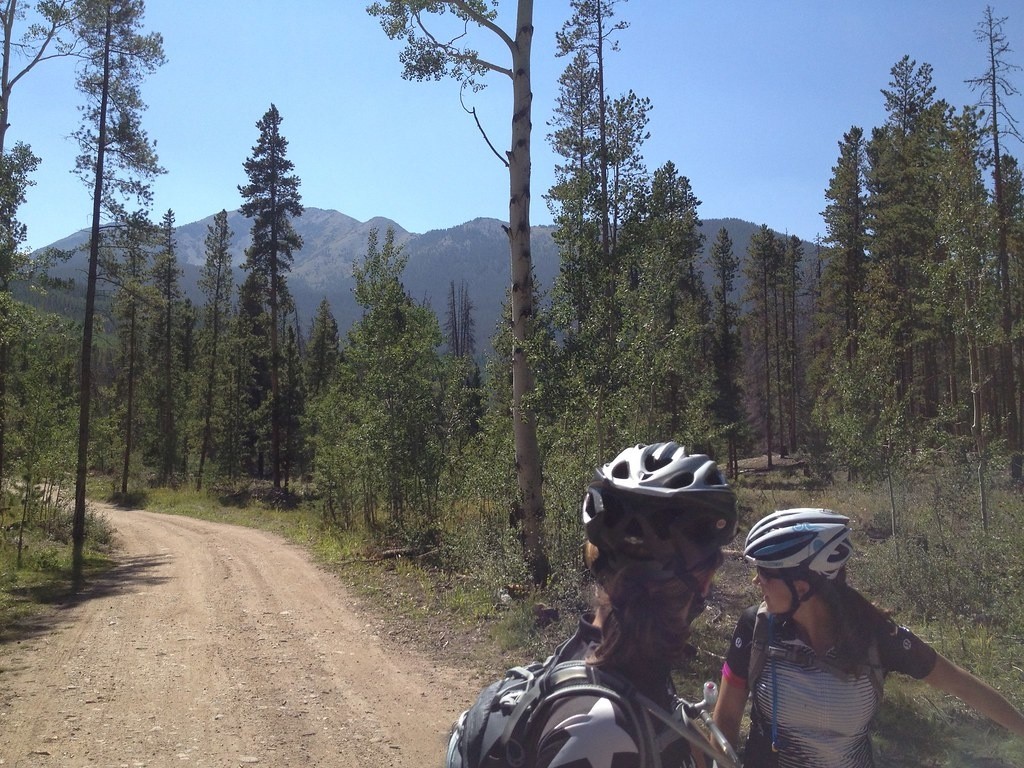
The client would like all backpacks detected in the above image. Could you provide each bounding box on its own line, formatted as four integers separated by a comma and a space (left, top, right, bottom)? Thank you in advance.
444, 641, 673, 768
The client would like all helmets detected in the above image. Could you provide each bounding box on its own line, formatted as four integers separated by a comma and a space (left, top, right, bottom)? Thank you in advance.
581, 442, 738, 560
743, 507, 854, 580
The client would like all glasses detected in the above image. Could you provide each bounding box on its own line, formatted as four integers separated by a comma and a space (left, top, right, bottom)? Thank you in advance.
756, 565, 784, 585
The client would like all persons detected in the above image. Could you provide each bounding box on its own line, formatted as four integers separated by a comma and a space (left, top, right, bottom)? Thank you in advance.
713, 507, 1024, 768
445, 439, 741, 768
